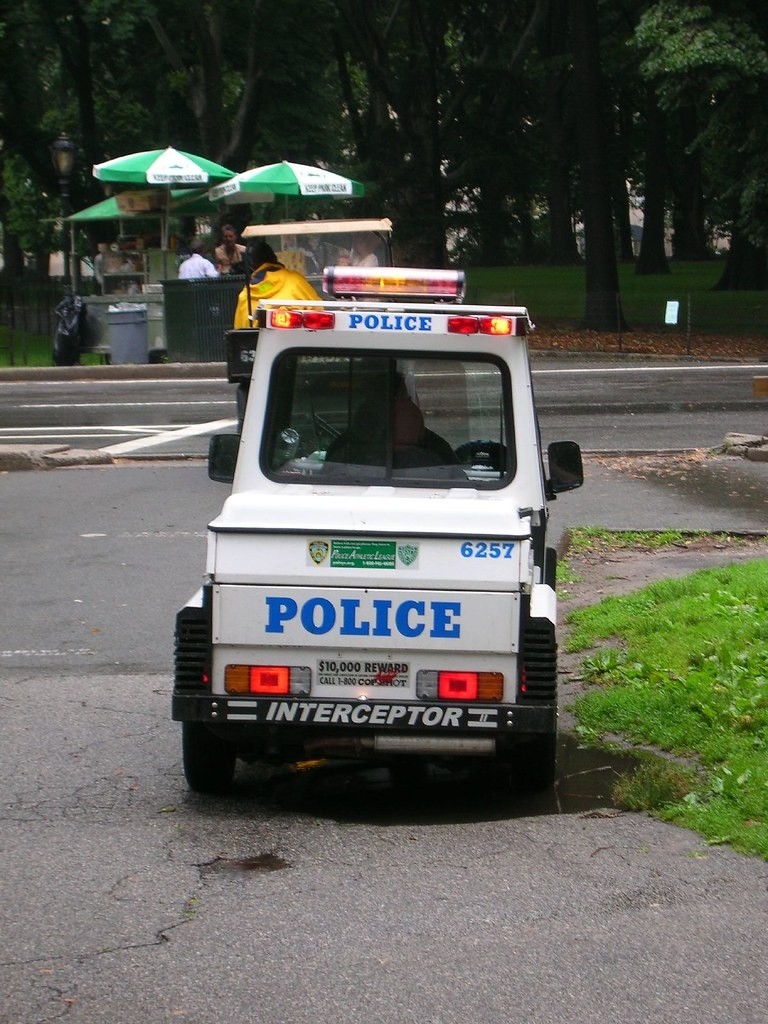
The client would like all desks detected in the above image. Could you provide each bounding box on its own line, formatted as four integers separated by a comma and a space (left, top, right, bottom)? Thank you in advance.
79, 294, 167, 363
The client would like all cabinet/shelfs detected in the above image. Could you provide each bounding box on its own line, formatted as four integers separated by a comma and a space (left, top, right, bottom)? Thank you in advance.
101, 249, 150, 293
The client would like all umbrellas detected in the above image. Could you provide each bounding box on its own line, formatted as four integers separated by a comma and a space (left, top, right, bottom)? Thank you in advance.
93, 148, 240, 248
60, 189, 235, 242
209, 159, 368, 220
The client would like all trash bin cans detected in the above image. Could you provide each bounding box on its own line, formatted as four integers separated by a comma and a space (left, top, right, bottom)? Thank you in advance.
107, 310, 148, 365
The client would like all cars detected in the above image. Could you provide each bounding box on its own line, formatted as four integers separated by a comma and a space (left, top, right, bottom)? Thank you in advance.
167, 261, 586, 797
248, 215, 396, 310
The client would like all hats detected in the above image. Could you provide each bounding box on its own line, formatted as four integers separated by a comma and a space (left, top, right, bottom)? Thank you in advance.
248, 242, 276, 267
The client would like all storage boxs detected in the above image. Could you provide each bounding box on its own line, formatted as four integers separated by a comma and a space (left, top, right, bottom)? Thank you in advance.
142, 285, 164, 294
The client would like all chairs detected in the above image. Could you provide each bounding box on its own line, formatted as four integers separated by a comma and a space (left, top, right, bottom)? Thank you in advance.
329, 442, 444, 466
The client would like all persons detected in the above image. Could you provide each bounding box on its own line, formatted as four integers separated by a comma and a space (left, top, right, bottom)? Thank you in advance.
328, 358, 468, 483
233, 240, 324, 329
178, 239, 219, 278
94, 241, 106, 292
336, 235, 378, 267
215, 225, 247, 275
